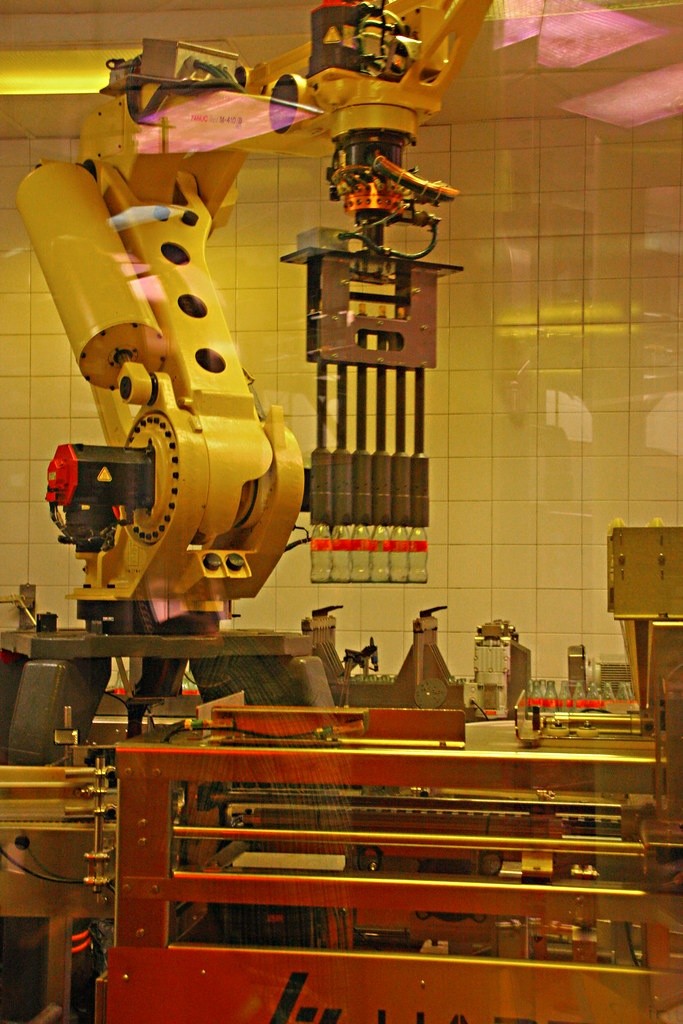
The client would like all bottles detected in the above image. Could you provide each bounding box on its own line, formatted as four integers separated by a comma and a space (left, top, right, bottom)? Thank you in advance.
351, 524, 371, 582
181, 672, 200, 696
391, 524, 408, 582
371, 525, 390, 582
310, 524, 331, 583
331, 525, 350, 583
410, 524, 428, 583
529, 678, 632, 716
114, 670, 127, 695
